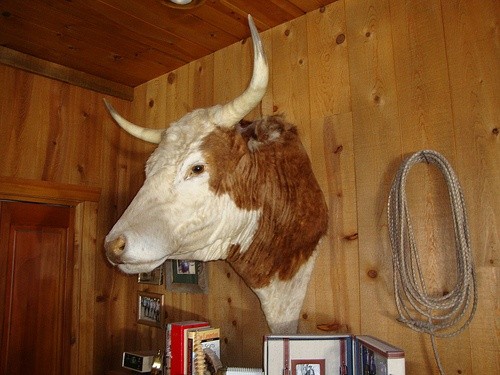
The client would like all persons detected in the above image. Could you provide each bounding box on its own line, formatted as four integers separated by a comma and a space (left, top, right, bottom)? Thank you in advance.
140, 260, 190, 321
305, 366, 315, 375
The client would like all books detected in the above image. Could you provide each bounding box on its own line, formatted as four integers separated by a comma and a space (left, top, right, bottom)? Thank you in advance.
166, 320, 221, 375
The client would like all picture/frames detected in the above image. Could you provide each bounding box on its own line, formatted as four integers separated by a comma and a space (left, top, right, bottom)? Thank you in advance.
165, 259, 208, 295
137, 265, 163, 285
291, 359, 325, 375
136, 291, 164, 328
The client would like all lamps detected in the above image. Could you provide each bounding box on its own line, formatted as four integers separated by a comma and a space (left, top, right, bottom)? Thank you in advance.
161, 0, 206, 10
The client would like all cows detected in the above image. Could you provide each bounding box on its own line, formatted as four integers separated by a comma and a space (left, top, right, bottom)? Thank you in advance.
102, 14, 329, 335
